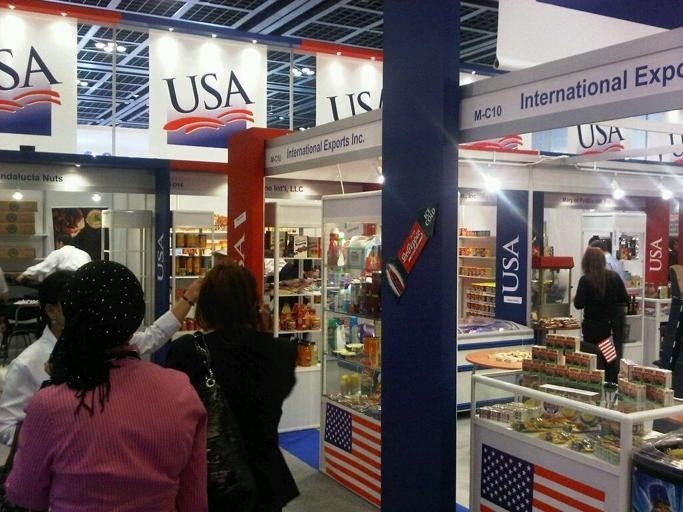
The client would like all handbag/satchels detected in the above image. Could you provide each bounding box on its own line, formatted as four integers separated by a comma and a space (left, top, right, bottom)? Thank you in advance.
202, 383, 259, 510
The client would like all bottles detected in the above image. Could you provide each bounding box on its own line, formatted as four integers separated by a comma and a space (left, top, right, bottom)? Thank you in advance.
327, 227, 346, 266
624, 295, 638, 315
621, 241, 632, 260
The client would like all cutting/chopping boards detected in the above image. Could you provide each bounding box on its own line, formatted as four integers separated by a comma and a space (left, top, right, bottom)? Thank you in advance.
466, 349, 533, 370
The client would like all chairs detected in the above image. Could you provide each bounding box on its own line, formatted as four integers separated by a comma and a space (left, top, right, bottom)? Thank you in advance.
1, 304, 42, 363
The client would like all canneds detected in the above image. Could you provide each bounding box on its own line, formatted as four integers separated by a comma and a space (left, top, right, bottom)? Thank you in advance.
170, 233, 210, 275
181, 318, 199, 330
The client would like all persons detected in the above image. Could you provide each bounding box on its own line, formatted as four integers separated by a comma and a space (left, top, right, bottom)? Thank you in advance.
1, 232, 300, 511
573, 235, 679, 383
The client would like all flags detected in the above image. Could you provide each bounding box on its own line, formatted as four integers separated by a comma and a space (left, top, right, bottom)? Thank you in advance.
480, 443, 605, 512
324, 402, 381, 509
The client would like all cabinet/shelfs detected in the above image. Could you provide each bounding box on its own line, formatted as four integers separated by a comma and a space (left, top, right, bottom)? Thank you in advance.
458, 233, 500, 318
470, 361, 681, 512
264, 204, 325, 369
171, 221, 227, 330
644, 296, 672, 360
321, 197, 383, 501
582, 226, 646, 339
